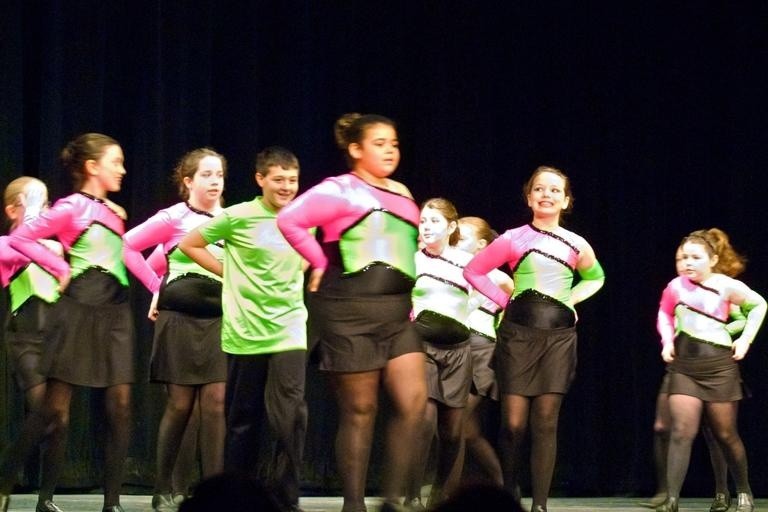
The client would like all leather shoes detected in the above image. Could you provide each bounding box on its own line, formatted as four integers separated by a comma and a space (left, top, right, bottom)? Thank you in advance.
35, 501, 61, 512
710, 492, 731, 511
151, 494, 178, 512
103, 506, 123, 511
656, 499, 678, 512
735, 491, 754, 512
0, 496, 10, 511
641, 494, 669, 508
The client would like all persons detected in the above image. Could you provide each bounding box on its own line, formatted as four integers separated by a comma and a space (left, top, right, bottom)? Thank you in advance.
463, 167, 604, 512
35, 133, 134, 512
641, 245, 734, 512
434, 218, 514, 512
145, 241, 168, 278
178, 150, 318, 506
0, 177, 66, 512
656, 228, 768, 512
121, 146, 228, 511
277, 113, 422, 512
411, 199, 496, 509
